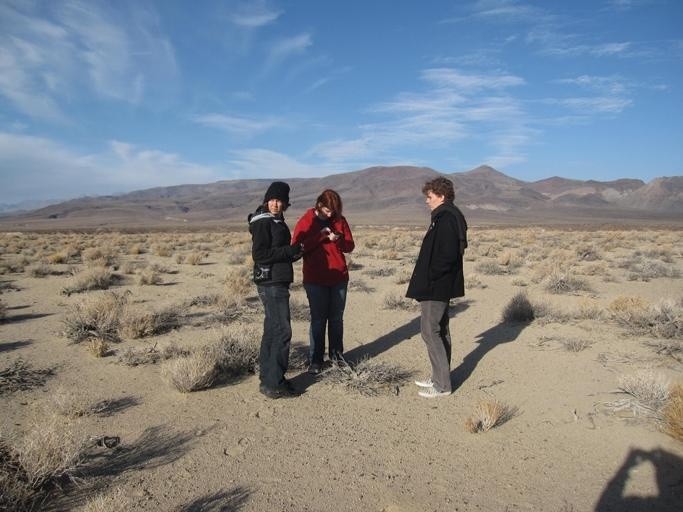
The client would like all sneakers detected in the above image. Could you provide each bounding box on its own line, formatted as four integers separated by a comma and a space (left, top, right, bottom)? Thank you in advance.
307, 357, 347, 375
258, 381, 302, 399
412, 377, 451, 397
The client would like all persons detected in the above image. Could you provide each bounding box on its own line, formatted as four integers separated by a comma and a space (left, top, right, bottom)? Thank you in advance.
405, 178, 468, 398
248, 182, 304, 399
291, 190, 354, 374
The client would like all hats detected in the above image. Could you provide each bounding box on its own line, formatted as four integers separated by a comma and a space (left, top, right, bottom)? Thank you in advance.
262, 180, 289, 211
